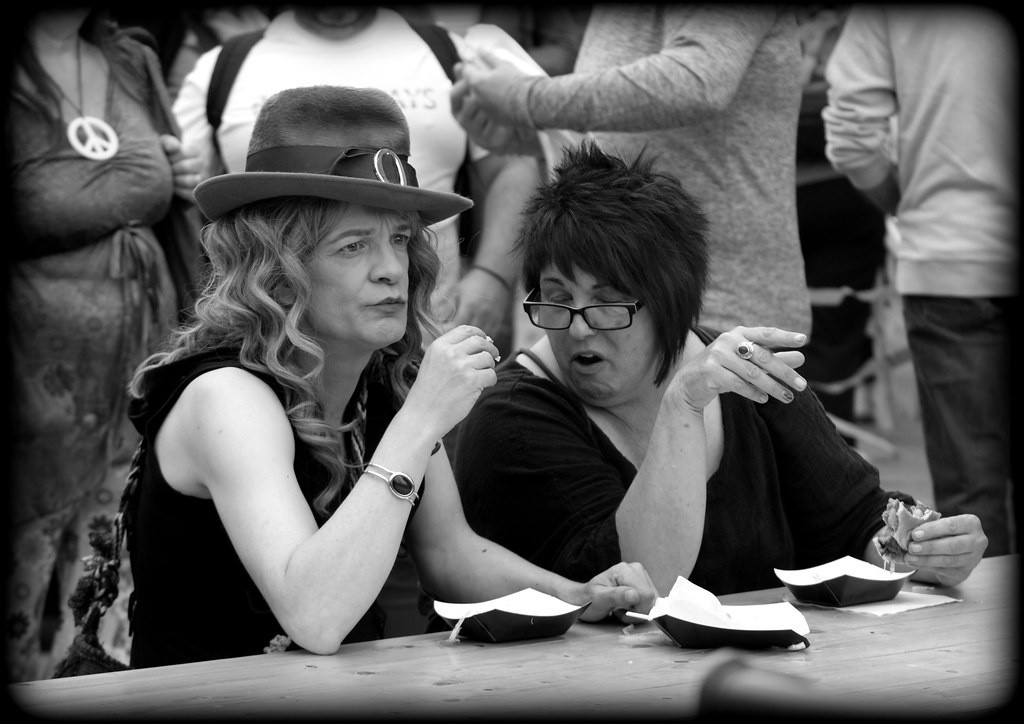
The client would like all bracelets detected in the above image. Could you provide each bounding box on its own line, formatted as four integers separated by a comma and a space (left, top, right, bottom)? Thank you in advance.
361, 461, 422, 509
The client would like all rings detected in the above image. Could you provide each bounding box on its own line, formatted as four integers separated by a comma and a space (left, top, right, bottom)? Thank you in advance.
735, 341, 754, 360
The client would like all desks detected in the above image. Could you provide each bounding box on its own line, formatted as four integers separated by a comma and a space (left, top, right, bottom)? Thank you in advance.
13, 553, 1018, 724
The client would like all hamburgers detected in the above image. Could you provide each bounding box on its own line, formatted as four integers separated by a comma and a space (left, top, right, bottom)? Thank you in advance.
872, 497, 943, 564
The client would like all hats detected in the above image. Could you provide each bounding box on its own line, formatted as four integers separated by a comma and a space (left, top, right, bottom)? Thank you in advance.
195, 86, 475, 225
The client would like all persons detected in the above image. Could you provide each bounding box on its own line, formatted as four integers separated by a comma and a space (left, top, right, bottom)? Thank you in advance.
820, 1, 1024, 561
2, 0, 199, 682
448, 0, 810, 386
161, 0, 549, 351
452, 138, 988, 633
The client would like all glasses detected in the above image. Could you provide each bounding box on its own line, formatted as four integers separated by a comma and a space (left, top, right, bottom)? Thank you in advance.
523, 289, 646, 330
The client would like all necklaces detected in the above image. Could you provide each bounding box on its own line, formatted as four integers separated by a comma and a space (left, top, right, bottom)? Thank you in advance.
35, 32, 120, 162
117, 85, 659, 675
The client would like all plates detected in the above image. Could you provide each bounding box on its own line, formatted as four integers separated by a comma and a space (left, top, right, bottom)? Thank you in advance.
649, 590, 811, 652
773, 555, 920, 607
433, 586, 594, 647
